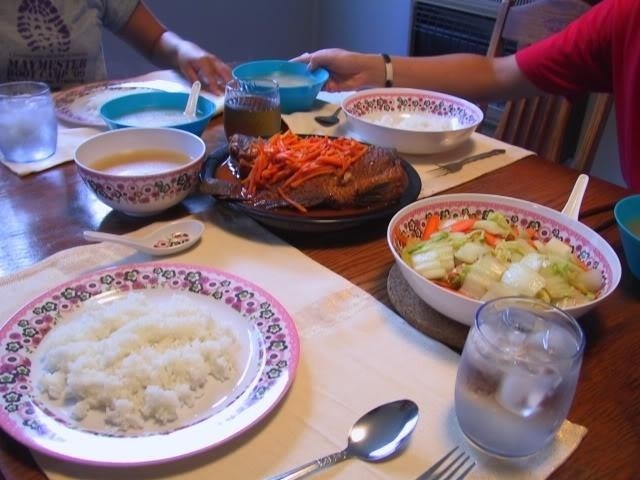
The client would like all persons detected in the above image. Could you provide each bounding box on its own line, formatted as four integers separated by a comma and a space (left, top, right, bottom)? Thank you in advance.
1, 0, 235, 97
286, 1, 640, 192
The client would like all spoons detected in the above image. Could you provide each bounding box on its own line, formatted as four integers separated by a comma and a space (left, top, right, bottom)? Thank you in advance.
315, 106, 342, 127
260, 399, 422, 478
559, 172, 589, 222
427, 149, 506, 176
84, 219, 205, 256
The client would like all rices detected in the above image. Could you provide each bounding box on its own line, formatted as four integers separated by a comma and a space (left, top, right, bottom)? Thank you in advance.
36, 291, 235, 429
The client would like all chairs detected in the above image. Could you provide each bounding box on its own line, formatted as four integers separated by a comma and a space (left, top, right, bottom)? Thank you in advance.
474, 0, 616, 173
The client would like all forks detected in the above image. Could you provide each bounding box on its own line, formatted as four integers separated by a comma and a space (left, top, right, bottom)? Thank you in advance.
416, 448, 476, 478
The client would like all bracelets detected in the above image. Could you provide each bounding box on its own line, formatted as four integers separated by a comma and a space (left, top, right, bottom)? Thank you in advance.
384, 51, 394, 90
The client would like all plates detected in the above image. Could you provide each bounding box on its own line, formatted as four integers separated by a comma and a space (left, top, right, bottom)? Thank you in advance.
55, 80, 185, 127
0, 262, 302, 469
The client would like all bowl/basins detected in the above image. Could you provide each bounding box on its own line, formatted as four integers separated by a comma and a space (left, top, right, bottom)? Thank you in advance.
100, 89, 214, 139
199, 133, 425, 235
231, 59, 330, 114
612, 194, 639, 277
391, 191, 620, 331
72, 127, 208, 214
341, 88, 483, 156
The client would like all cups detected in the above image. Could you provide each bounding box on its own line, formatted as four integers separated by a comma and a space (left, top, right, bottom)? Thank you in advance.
0, 81, 60, 164
225, 78, 284, 137
458, 294, 583, 459
